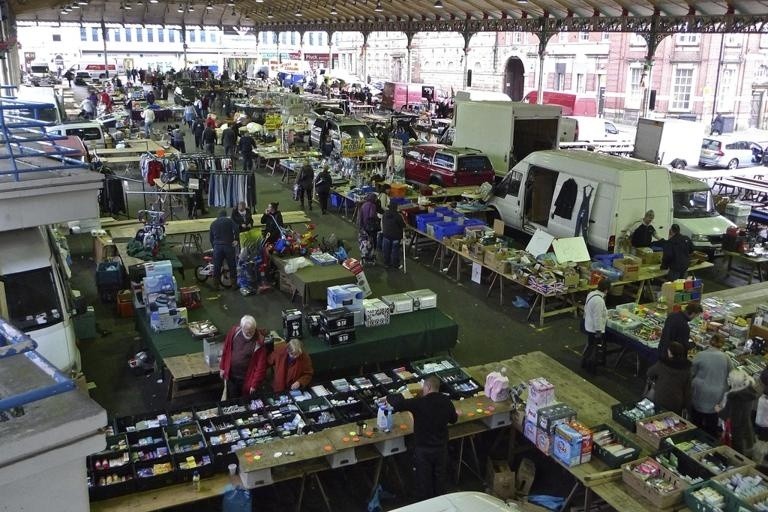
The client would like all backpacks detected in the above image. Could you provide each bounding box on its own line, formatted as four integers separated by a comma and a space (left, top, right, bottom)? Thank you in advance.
375, 199, 385, 214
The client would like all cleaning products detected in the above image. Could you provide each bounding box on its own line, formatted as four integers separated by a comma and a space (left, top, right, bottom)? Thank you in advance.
377, 402, 395, 431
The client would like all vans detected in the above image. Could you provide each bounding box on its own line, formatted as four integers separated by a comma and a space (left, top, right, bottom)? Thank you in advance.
0, 222, 84, 380
15, 86, 130, 167
192, 64, 219, 77
276, 71, 305, 90
30, 60, 118, 82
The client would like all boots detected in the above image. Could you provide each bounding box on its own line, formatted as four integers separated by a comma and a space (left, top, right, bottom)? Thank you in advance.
213, 277, 239, 290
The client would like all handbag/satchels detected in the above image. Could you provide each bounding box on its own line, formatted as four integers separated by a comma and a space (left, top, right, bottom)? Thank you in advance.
366, 217, 381, 233
293, 182, 301, 201
279, 224, 295, 239
314, 172, 325, 187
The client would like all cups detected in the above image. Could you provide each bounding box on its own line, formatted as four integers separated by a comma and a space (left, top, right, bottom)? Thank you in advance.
356, 421, 367, 436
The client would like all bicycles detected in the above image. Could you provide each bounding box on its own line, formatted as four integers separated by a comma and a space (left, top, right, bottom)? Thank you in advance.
194, 256, 233, 288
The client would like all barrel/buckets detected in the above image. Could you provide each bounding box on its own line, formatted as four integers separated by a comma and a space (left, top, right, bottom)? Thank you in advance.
490, 377, 510, 403
483, 371, 502, 398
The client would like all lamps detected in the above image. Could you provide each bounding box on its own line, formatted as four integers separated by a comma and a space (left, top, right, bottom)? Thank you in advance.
266, 0, 444, 19
177, 0, 235, 14
60, 0, 88, 15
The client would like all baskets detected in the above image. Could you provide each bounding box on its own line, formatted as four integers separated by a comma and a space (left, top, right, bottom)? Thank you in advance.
416, 206, 489, 242
588, 396, 768, 512
94, 261, 119, 305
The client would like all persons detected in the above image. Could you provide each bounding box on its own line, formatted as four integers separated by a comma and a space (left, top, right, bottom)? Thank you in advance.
382, 375, 458, 502
647, 302, 767, 461
220, 315, 313, 397
710, 113, 724, 135
583, 280, 613, 368
64, 65, 455, 296
625, 210, 747, 281
